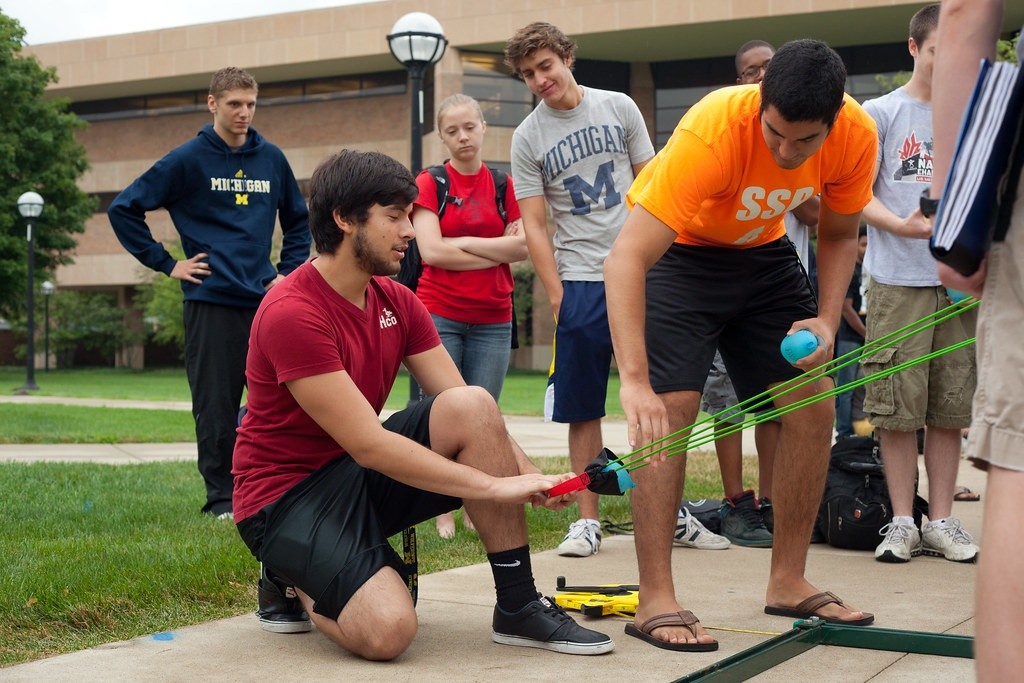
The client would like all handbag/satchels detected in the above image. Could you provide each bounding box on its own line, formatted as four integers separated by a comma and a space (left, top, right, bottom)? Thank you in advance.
680, 499, 723, 535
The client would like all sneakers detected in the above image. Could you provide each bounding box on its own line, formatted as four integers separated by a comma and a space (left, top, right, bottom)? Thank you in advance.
720, 490, 773, 547
760, 497, 817, 542
875, 519, 922, 564
924, 517, 979, 562
557, 519, 602, 557
490, 592, 615, 654
258, 561, 312, 633
672, 507, 731, 550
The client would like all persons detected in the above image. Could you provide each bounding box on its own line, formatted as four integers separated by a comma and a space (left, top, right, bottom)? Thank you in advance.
235, 147, 616, 660
604, 39, 882, 651
109, 0, 1024, 683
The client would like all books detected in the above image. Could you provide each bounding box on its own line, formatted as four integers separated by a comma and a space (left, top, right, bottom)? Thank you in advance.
928, 56, 1023, 279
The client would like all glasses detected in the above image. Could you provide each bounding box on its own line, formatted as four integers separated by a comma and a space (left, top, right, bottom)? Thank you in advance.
740, 63, 770, 79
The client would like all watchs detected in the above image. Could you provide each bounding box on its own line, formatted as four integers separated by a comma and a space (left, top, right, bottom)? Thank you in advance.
920, 187, 944, 221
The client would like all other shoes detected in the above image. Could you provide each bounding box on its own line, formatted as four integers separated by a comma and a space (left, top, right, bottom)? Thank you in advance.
211, 504, 234, 522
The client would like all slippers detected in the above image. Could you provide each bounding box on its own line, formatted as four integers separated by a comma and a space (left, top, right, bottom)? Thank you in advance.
953, 486, 980, 501
764, 592, 874, 625
625, 610, 718, 651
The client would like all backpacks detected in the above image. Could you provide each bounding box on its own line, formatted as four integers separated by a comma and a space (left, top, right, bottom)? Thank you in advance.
398, 158, 508, 294
817, 438, 929, 551
